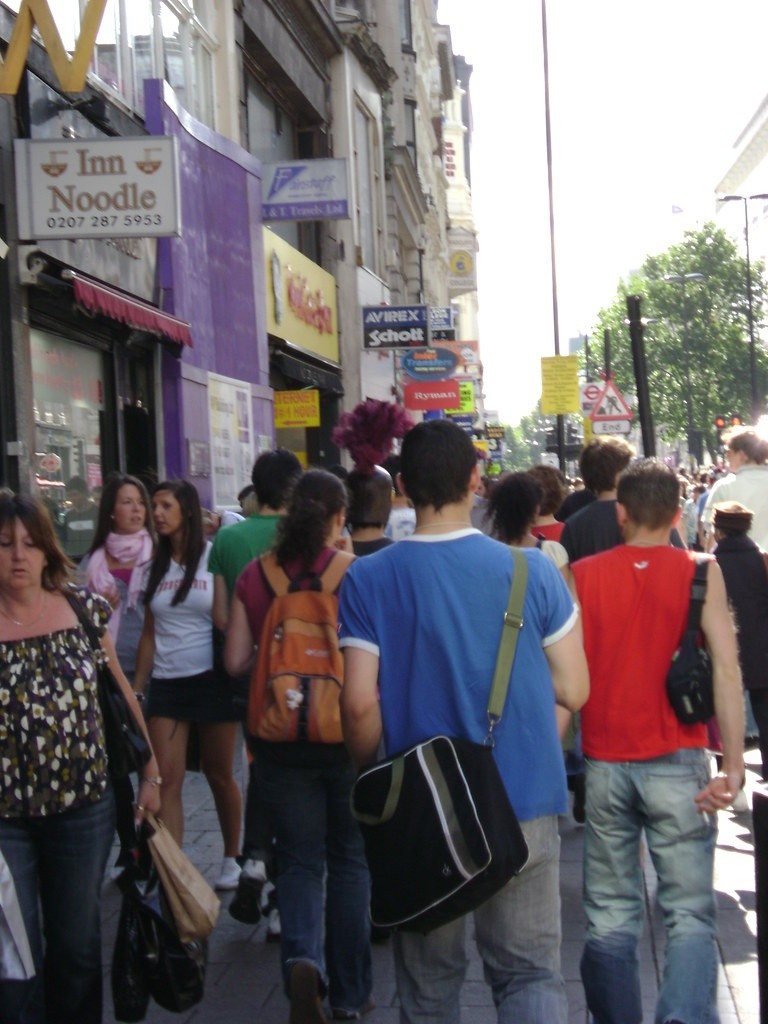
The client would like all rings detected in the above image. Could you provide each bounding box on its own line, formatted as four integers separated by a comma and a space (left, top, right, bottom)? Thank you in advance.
137, 805, 144, 811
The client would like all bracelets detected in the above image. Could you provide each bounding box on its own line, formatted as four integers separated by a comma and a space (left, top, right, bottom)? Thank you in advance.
133, 691, 145, 702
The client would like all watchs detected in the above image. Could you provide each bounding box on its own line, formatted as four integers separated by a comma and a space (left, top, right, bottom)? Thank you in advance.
142, 776, 162, 785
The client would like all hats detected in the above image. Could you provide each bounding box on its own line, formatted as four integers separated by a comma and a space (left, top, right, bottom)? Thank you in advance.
712, 502, 754, 532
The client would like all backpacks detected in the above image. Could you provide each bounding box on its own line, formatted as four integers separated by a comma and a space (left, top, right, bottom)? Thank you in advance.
247, 548, 356, 741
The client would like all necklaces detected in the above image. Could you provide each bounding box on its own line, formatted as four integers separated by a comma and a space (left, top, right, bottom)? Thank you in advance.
627, 539, 661, 546
0, 590, 48, 628
416, 521, 471, 530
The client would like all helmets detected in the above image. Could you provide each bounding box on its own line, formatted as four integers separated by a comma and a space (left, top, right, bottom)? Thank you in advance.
331, 397, 417, 525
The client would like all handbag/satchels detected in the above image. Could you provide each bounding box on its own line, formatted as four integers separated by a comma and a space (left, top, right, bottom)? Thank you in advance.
0, 851, 35, 979
109, 807, 221, 1024
664, 554, 713, 724
62, 588, 154, 773
356, 736, 531, 936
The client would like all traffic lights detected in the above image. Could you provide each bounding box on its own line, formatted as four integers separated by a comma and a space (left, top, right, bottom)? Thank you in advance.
715, 416, 725, 453
546, 429, 558, 454
566, 423, 577, 462
731, 414, 741, 427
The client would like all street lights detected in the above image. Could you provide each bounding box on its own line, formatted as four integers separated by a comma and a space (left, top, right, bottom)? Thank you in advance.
718, 192, 767, 424
663, 271, 705, 476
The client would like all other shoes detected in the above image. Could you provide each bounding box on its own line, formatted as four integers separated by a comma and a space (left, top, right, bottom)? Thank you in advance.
228, 858, 267, 924
731, 788, 749, 814
572, 772, 587, 823
289, 960, 328, 1024
331, 993, 376, 1024
267, 908, 282, 938
215, 856, 242, 890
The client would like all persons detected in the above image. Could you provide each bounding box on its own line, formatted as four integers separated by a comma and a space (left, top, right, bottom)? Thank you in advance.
224, 467, 377, 1024
323, 455, 417, 556
554, 457, 745, 1024
672, 461, 730, 553
700, 431, 768, 559
206, 447, 304, 944
469, 442, 689, 826
337, 420, 591, 1024
0, 486, 164, 1023
74, 473, 243, 890
708, 501, 768, 811
